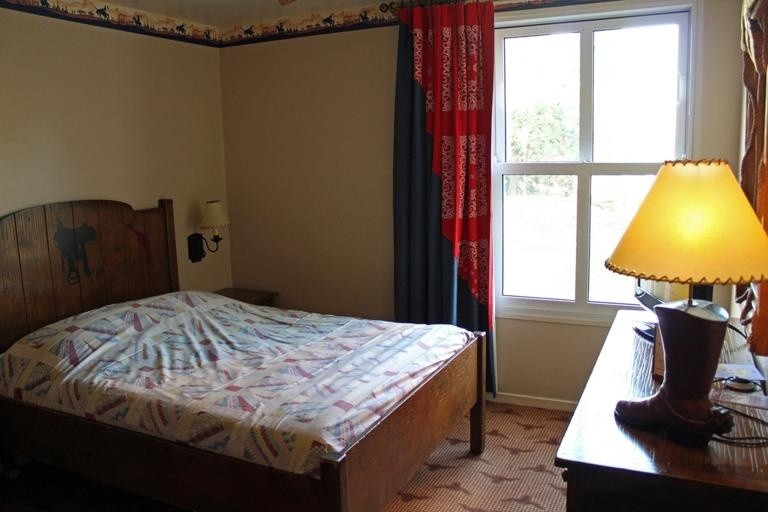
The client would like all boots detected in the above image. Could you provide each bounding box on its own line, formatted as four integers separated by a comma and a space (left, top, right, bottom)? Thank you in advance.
613, 297, 730, 452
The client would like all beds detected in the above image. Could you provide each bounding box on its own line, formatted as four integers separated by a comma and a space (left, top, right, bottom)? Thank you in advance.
0, 196, 488, 512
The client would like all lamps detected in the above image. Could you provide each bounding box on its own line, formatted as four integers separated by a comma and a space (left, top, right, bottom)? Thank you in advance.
604, 156, 768, 451
188, 199, 230, 268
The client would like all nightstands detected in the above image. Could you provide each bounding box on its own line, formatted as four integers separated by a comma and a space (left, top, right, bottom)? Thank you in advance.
204, 288, 279, 308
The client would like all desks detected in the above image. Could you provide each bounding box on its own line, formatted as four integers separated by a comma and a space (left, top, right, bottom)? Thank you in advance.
552, 307, 768, 512
